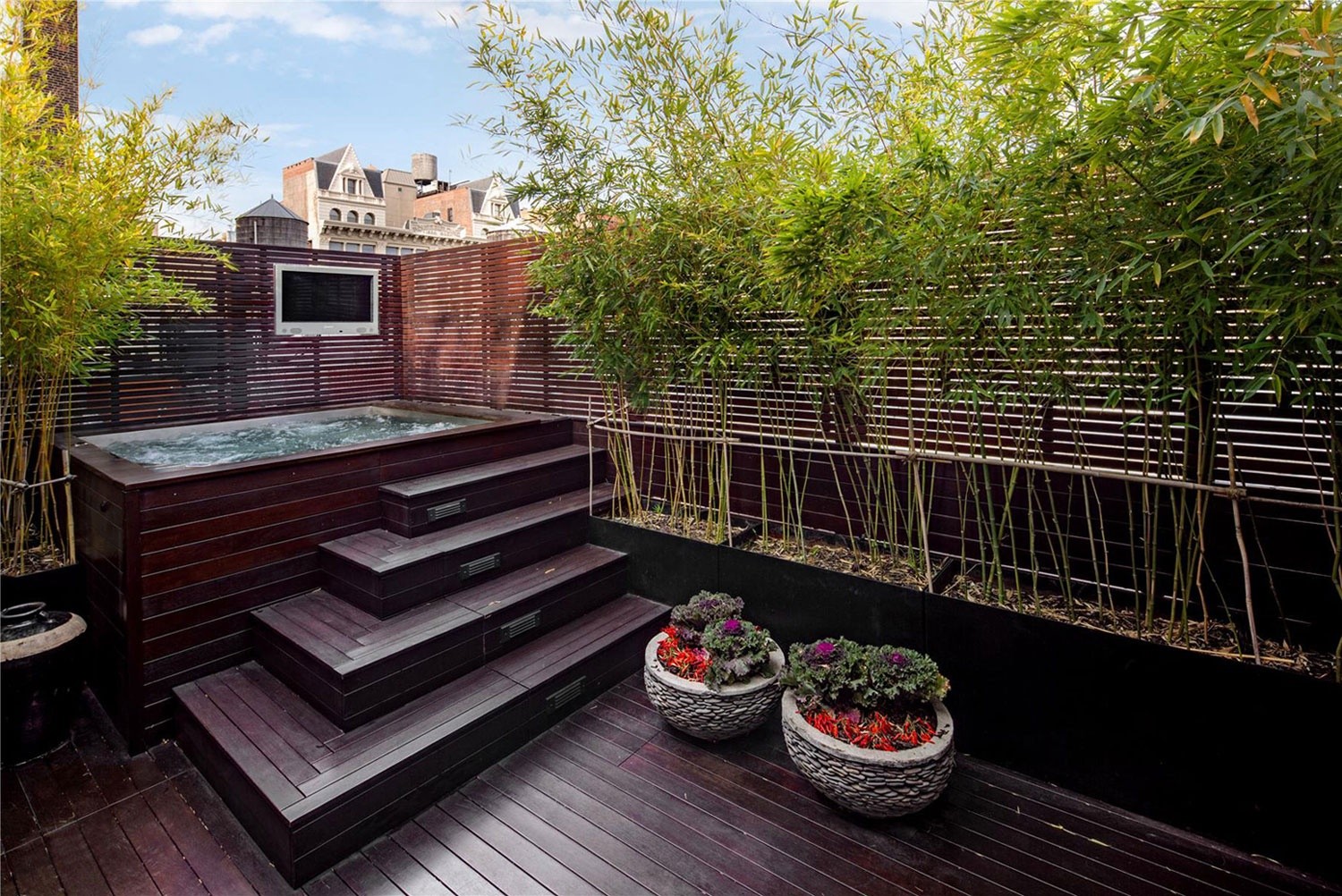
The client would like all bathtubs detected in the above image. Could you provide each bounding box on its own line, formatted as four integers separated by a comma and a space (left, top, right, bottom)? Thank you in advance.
79, 403, 495, 478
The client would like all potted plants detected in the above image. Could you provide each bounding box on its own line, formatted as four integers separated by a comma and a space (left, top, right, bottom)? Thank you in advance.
774, 634, 957, 818
644, 589, 786, 743
